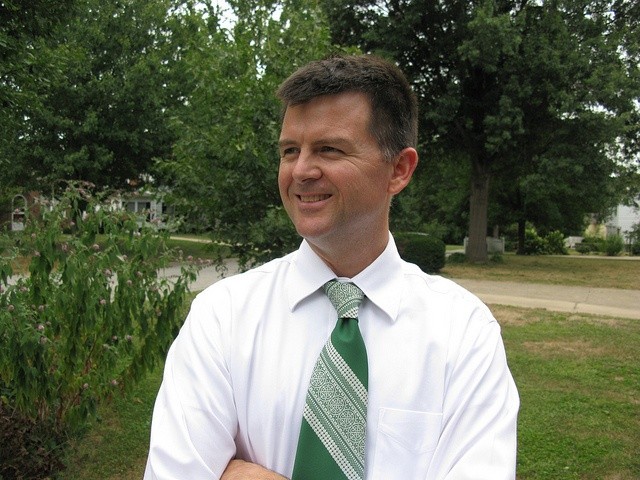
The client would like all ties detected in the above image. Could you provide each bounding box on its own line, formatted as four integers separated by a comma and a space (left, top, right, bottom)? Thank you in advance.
292, 280, 368, 480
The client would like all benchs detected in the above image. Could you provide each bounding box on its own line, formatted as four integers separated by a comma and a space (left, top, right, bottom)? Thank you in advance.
463, 235, 506, 254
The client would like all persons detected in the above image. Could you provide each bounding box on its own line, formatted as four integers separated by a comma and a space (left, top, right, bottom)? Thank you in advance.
143, 53, 521, 480
